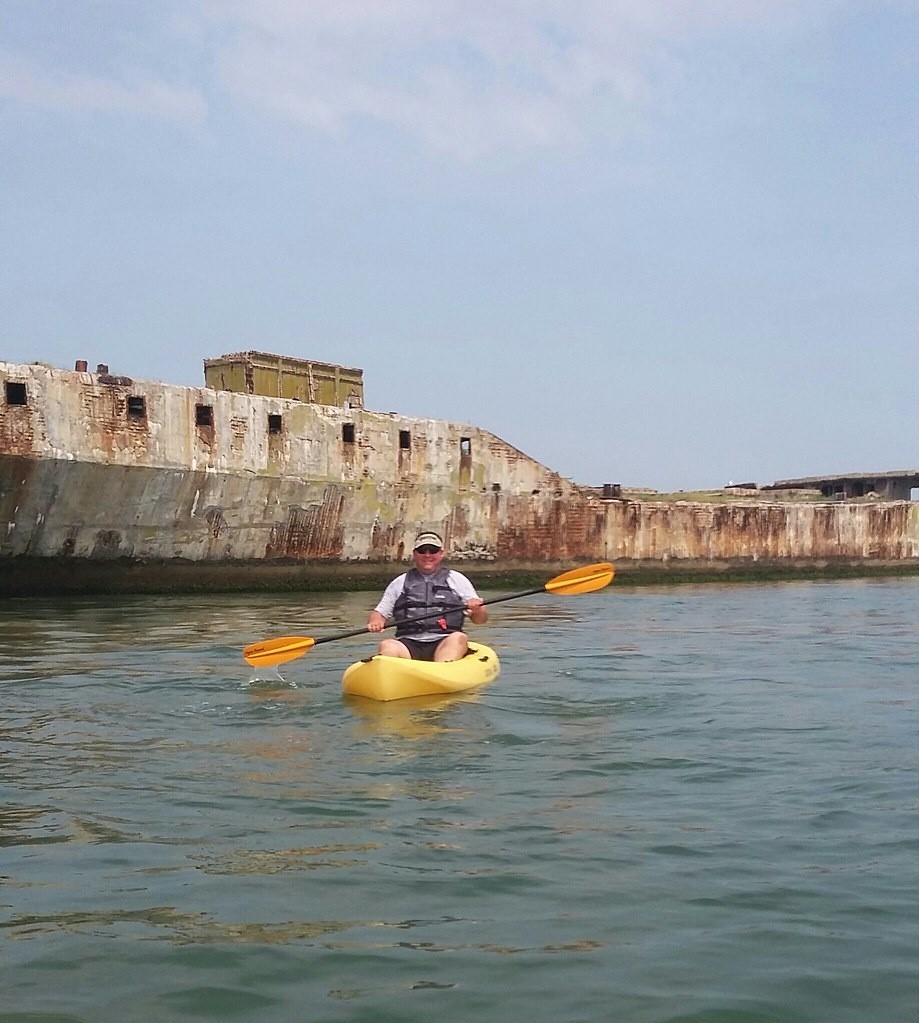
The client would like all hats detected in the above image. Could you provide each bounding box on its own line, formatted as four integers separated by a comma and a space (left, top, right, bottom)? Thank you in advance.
413, 534, 444, 551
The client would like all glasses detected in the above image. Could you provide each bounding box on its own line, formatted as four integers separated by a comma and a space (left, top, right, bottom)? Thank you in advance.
415, 546, 441, 554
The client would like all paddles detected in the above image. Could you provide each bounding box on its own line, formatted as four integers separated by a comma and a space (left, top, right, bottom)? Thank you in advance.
242, 561, 616, 668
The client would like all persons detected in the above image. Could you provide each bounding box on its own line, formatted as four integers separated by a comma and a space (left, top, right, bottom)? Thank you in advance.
367, 531, 490, 663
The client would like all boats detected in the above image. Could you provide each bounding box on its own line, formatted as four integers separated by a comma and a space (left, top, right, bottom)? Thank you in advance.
342, 641, 500, 702
